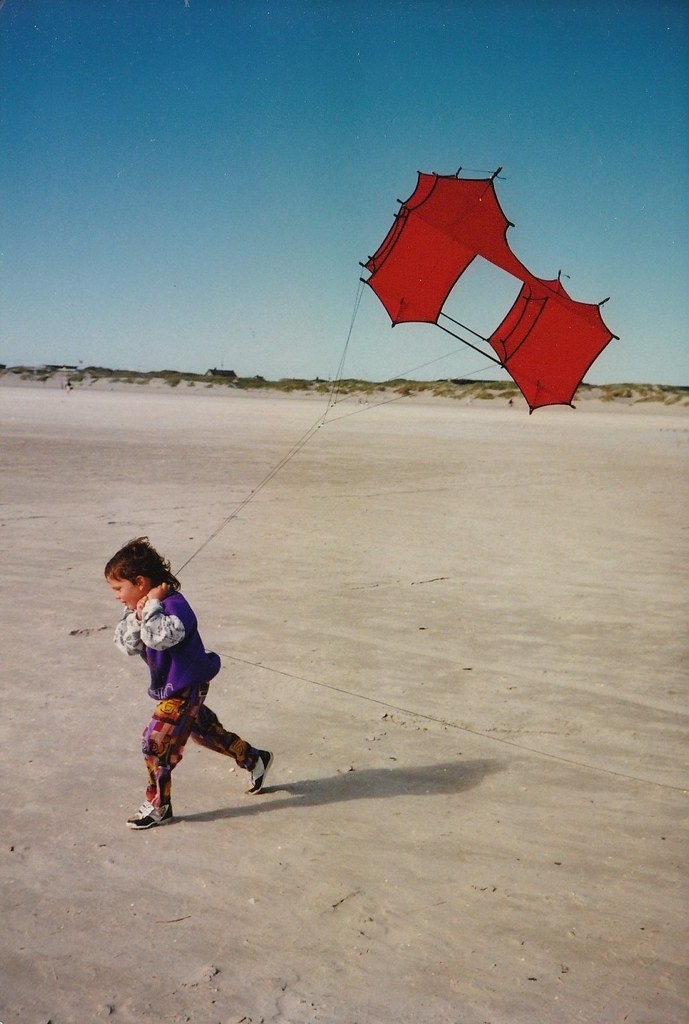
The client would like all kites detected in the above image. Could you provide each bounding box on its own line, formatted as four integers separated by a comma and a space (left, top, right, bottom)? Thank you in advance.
359, 165, 620, 415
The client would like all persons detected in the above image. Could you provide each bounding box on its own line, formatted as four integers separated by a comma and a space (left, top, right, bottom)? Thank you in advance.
105, 535, 274, 830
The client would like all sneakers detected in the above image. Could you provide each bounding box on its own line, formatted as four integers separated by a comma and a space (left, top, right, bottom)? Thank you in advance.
127, 800, 173, 829
245, 750, 274, 795
